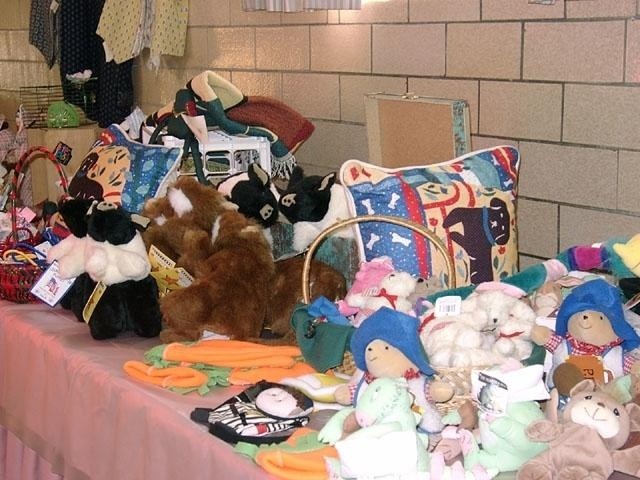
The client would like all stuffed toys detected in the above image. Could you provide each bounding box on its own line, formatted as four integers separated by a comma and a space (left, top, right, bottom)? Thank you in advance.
190, 232, 638, 480
32, 162, 350, 346
31, 162, 350, 345
188, 233, 640, 479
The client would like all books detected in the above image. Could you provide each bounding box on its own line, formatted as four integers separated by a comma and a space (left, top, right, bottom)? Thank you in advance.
365, 87, 472, 173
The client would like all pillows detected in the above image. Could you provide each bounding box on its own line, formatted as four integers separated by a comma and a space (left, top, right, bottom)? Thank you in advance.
337, 141, 535, 288
68, 122, 184, 214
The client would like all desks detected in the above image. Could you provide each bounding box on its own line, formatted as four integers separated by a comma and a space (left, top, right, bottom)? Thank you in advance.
0, 295, 328, 479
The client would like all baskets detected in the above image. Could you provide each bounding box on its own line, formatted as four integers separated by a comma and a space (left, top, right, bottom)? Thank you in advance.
301, 216, 457, 376
21, 80, 98, 128
0, 148, 71, 304
